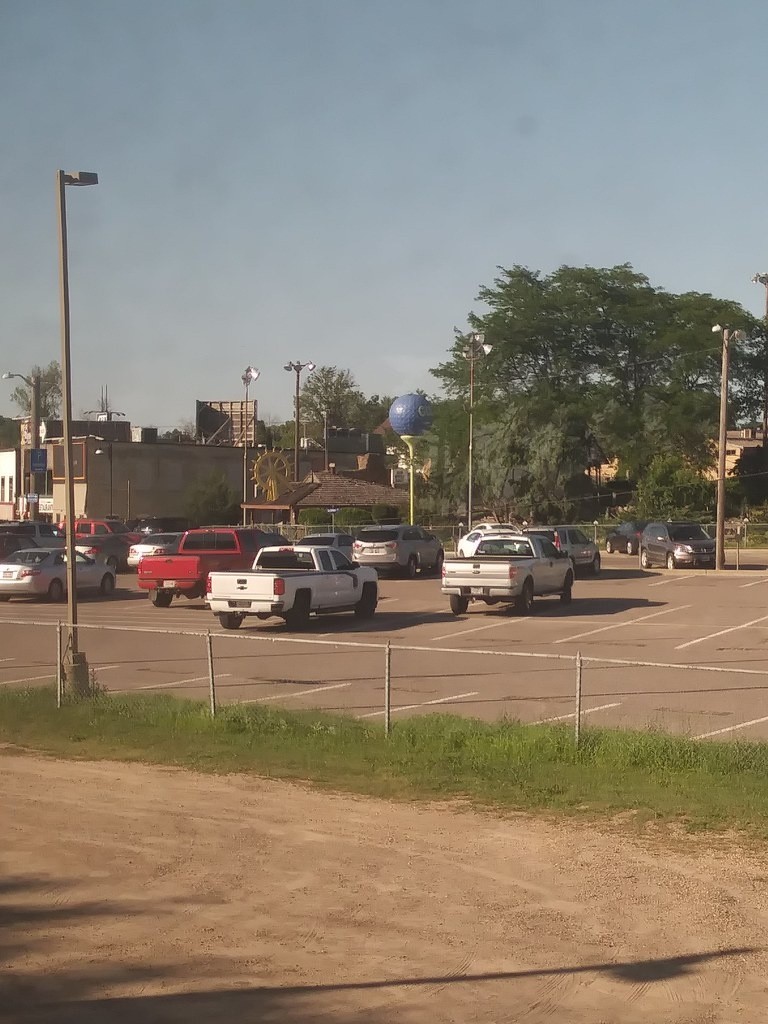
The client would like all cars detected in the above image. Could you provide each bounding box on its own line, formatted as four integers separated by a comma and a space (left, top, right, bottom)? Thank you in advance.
458, 521, 521, 557
0, 547, 117, 601
126, 530, 186, 568
294, 531, 356, 562
264, 530, 294, 546
604, 521, 653, 554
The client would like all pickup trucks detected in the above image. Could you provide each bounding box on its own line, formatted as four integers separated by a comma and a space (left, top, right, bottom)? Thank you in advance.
136, 526, 275, 607
204, 544, 379, 630
441, 533, 575, 616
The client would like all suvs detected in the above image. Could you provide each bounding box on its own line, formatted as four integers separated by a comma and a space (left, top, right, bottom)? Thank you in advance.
641, 521, 725, 570
522, 524, 601, 575
0, 515, 200, 573
351, 524, 445, 580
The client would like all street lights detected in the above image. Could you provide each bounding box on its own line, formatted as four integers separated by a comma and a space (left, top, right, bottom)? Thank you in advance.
239, 365, 261, 528
53, 165, 98, 653
283, 359, 317, 481
712, 321, 748, 569
2, 366, 44, 520
463, 330, 493, 531
